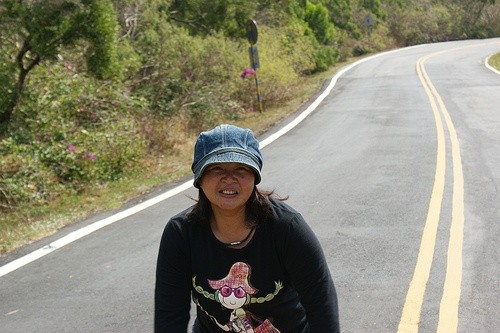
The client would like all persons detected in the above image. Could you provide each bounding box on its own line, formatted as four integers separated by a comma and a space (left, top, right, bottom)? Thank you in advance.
154, 123, 342, 333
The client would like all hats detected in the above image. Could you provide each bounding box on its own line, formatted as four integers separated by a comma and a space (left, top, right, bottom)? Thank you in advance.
190, 123, 262, 186
